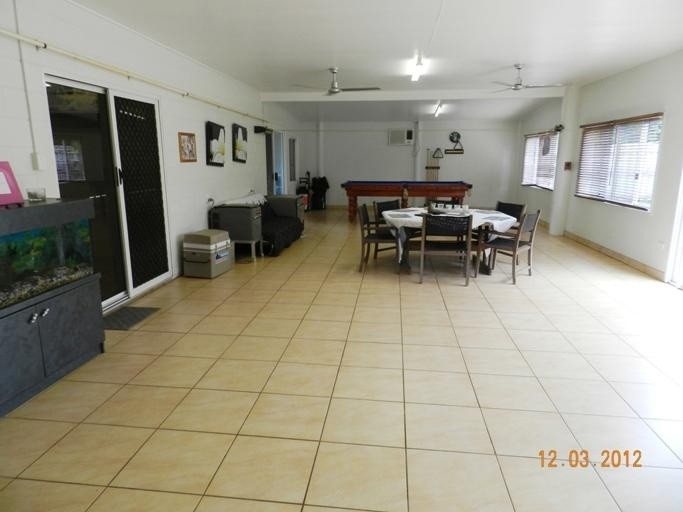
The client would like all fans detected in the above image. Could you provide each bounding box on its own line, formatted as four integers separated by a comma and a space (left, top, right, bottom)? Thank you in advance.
288, 68, 382, 97
485, 63, 566, 92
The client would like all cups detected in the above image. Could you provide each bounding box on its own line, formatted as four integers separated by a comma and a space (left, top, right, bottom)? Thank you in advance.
25, 187, 46, 201
430, 202, 469, 213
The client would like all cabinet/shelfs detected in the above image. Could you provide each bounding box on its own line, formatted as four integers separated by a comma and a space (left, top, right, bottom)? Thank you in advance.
207, 203, 263, 264
0, 277, 104, 415
266, 195, 304, 224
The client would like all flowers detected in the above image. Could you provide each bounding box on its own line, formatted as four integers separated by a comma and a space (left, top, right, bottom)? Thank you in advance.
233, 128, 246, 161
209, 129, 224, 165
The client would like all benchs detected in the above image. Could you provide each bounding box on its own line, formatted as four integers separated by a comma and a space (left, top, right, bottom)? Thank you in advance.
224, 194, 303, 256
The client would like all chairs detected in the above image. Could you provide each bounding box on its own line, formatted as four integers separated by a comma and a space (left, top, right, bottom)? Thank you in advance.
355, 198, 542, 287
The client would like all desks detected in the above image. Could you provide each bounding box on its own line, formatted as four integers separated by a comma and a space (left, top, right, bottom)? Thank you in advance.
339, 179, 472, 223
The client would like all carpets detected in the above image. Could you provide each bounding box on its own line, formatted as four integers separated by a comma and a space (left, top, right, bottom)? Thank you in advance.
103, 306, 162, 331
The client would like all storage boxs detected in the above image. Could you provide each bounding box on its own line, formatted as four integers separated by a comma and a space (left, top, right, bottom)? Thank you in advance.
182, 228, 230, 263
181, 241, 234, 282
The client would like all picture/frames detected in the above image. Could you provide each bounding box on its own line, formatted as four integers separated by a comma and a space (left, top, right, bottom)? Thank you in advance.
204, 120, 226, 168
176, 131, 196, 163
0, 160, 24, 209
230, 122, 248, 164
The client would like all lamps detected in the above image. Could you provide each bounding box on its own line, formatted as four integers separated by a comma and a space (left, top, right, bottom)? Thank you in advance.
432, 103, 442, 119
405, 53, 423, 84
